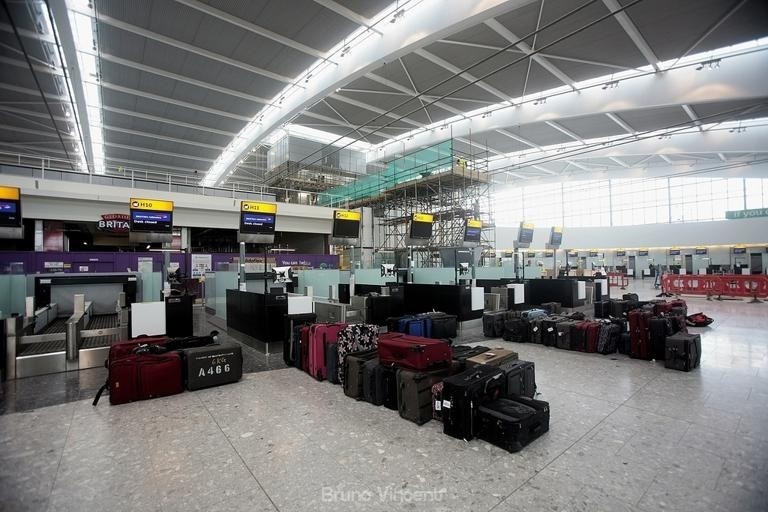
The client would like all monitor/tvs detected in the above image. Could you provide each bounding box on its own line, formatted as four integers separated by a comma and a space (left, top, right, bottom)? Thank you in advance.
464, 219, 482, 242
381, 264, 396, 278
333, 210, 360, 238
410, 213, 434, 239
519, 222, 535, 243
130, 198, 173, 234
0, 186, 21, 228
459, 262, 470, 276
272, 266, 293, 284
551, 226, 562, 245
240, 201, 277, 235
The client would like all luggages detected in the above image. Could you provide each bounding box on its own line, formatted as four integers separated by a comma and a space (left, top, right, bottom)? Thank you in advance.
283, 312, 550, 454
483, 292, 714, 372
106, 330, 243, 404
